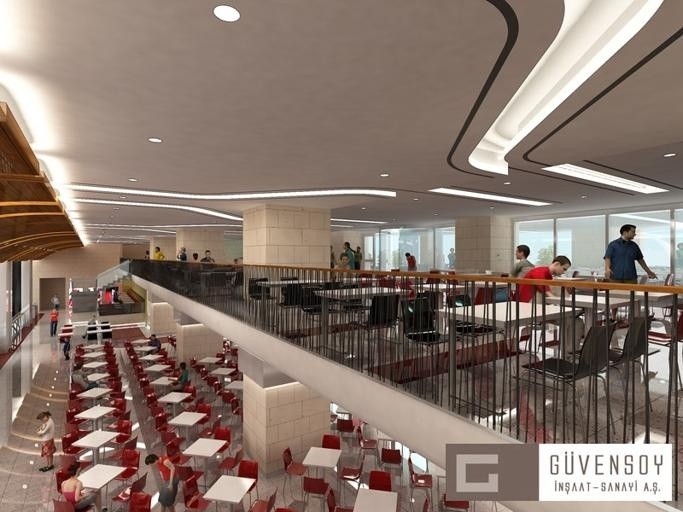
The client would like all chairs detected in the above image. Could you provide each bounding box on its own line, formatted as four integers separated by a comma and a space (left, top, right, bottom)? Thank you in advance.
248, 271, 682, 412
278, 413, 498, 512
159, 262, 242, 303
152, 341, 278, 512
52, 341, 152, 512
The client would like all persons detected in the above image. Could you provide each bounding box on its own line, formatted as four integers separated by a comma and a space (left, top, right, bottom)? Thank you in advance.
447, 248, 455, 268
603, 224, 658, 321
519, 255, 584, 359
495, 245, 535, 303
330, 242, 362, 278
38, 286, 189, 512
145, 246, 215, 263
405, 252, 417, 271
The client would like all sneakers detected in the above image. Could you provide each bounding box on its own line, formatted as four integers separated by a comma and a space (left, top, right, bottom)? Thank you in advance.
39, 465, 54, 472
